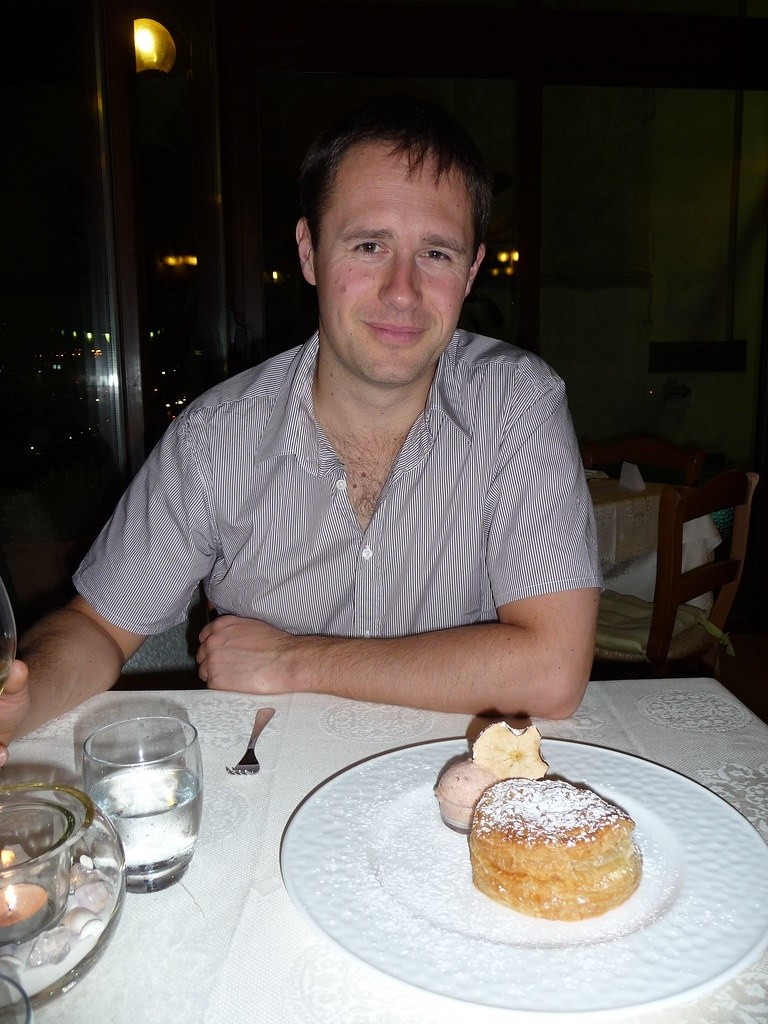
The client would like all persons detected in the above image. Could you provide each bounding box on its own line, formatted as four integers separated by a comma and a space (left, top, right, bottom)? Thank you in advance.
0, 94, 605, 766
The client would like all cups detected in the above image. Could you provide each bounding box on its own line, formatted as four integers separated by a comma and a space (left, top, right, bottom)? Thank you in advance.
82, 716, 204, 894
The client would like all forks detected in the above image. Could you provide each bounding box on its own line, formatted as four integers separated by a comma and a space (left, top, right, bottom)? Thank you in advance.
226, 708, 276, 775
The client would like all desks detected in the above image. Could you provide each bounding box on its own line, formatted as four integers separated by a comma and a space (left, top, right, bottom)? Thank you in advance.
589, 476, 724, 633
0, 674, 767, 1021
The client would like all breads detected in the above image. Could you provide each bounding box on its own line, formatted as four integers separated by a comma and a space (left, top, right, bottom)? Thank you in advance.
468, 778, 643, 922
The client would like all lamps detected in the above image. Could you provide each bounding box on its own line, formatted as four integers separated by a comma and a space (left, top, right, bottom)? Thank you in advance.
134, 17, 176, 85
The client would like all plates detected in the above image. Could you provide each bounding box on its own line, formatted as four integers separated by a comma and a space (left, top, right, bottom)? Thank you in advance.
281, 739, 768, 1018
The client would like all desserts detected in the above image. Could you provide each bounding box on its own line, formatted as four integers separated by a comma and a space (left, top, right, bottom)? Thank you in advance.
433, 721, 549, 834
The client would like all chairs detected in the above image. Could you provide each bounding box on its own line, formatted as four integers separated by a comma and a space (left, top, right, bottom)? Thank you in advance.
584, 430, 715, 485
3, 526, 207, 661
599, 468, 760, 680
